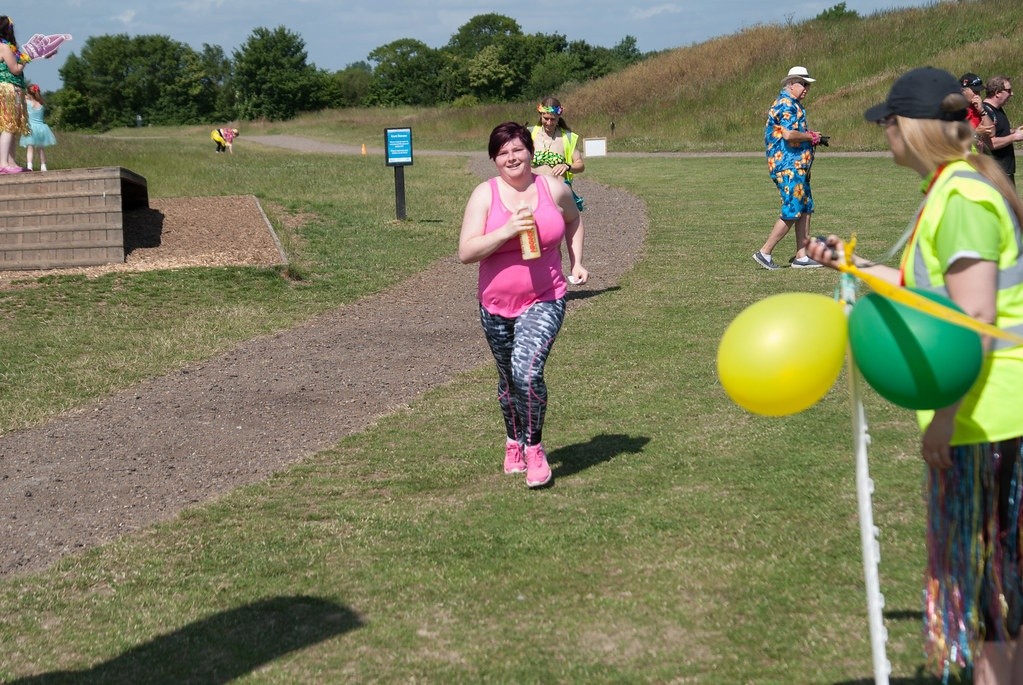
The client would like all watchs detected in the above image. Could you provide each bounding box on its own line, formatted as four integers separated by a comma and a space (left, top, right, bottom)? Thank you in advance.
564, 162, 571, 171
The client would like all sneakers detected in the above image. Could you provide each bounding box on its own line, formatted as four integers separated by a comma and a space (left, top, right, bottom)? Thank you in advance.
503, 440, 527, 474
526, 444, 552, 486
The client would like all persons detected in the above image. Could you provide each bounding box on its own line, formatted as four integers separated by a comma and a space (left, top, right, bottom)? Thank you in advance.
526, 97, 584, 215
752, 66, 822, 271
0, 16, 31, 175
458, 122, 590, 488
211, 128, 239, 154
803, 65, 1023, 684
959, 73, 1023, 189
19, 84, 57, 171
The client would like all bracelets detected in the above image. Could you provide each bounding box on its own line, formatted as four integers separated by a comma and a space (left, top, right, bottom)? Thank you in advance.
979, 111, 987, 116
806, 131, 818, 145
979, 109, 985, 114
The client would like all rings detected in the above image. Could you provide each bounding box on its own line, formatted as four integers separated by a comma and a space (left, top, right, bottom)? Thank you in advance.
932, 453, 939, 458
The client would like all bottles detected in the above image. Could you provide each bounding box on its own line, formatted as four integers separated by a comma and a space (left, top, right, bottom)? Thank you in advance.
361, 143, 366, 154
518, 207, 541, 260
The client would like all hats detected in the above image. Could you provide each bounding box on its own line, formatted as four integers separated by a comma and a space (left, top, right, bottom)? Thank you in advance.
959, 72, 985, 91
864, 67, 969, 122
781, 66, 817, 83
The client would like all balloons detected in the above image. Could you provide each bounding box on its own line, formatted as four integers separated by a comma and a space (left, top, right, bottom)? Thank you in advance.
711, 292, 849, 420
849, 283, 986, 413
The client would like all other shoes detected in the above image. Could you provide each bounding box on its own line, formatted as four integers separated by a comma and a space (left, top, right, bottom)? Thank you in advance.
791, 257, 824, 269
752, 250, 782, 269
0, 165, 32, 174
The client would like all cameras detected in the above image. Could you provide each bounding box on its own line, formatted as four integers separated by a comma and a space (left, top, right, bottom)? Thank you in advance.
818, 136, 830, 146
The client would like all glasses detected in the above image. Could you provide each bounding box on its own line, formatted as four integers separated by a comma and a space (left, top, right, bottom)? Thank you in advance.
795, 81, 811, 86
877, 117, 896, 127
1003, 89, 1011, 93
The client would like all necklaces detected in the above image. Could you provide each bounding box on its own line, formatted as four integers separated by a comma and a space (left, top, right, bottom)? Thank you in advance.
542, 124, 556, 151
2, 39, 30, 63
